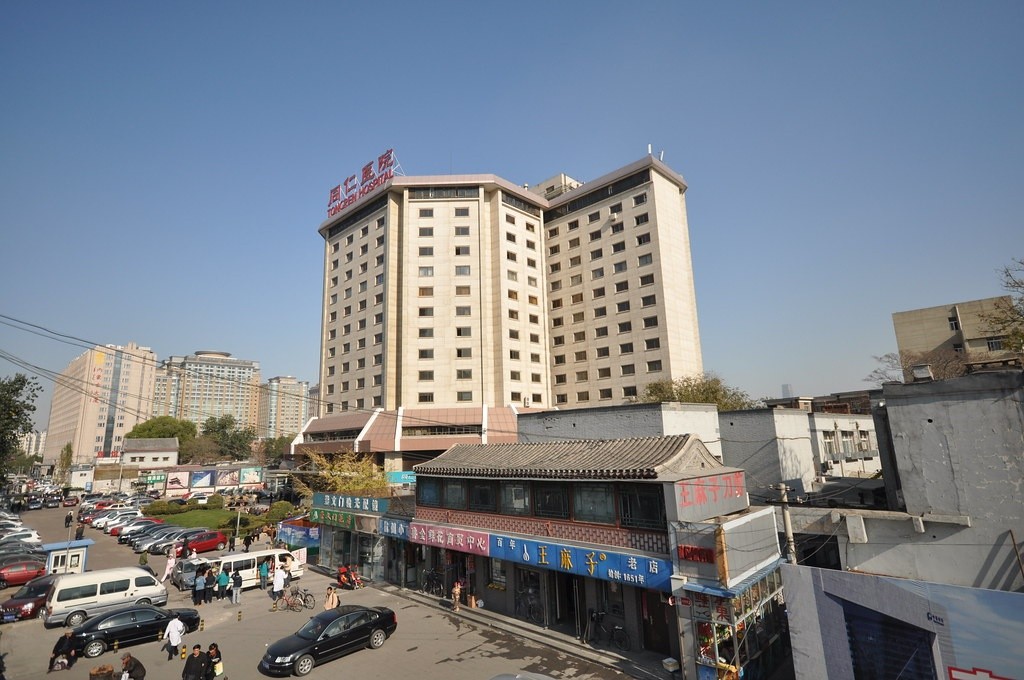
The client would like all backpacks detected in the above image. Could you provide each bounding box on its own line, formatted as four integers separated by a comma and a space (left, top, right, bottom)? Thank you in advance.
332, 593, 340, 608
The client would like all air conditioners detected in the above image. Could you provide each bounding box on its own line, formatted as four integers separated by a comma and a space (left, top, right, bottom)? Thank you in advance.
525, 397, 530, 407
859, 442, 867, 450
824, 459, 834, 470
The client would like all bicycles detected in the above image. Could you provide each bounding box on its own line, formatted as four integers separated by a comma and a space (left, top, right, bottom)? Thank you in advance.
278, 581, 323, 613
421, 568, 444, 598
517, 588, 546, 624
591, 609, 633, 654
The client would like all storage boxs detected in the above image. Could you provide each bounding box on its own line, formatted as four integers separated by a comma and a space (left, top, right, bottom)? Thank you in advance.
663, 657, 679, 672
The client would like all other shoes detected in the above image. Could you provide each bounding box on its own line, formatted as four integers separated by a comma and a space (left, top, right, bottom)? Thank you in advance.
232, 602, 241, 605
194, 604, 201, 607
216, 597, 226, 602
66, 666, 71, 670
175, 653, 178, 656
168, 658, 173, 661
203, 601, 212, 605
46, 669, 53, 674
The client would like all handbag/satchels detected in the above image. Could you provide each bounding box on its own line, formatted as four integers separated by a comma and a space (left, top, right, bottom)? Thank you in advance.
121, 671, 129, 680
214, 661, 224, 677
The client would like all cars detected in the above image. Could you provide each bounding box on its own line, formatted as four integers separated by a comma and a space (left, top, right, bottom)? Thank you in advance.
259, 604, 398, 678
174, 530, 228, 556
0, 561, 45, 590
66, 603, 202, 659
0, 573, 71, 622
171, 558, 202, 592
153, 527, 211, 555
0, 474, 268, 566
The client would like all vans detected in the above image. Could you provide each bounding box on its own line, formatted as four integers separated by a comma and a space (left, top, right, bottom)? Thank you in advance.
43, 566, 168, 627
190, 549, 304, 595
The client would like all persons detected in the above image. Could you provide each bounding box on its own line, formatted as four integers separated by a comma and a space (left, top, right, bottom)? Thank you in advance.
247, 506, 268, 515
187, 548, 197, 559
182, 644, 211, 680
168, 544, 177, 564
120, 651, 146, 680
451, 577, 466, 611
194, 564, 216, 606
273, 555, 292, 605
163, 612, 184, 660
46, 628, 77, 673
253, 527, 260, 541
75, 525, 83, 540
138, 550, 148, 565
179, 535, 188, 559
160, 553, 175, 583
243, 532, 253, 552
263, 523, 286, 550
287, 513, 292, 518
336, 563, 360, 590
258, 559, 269, 590
215, 568, 242, 604
229, 535, 236, 552
323, 587, 338, 611
65, 509, 73, 527
206, 642, 221, 680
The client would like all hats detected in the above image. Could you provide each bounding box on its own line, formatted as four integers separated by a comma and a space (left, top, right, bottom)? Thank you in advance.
120, 652, 131, 659
65, 627, 74, 633
168, 554, 173, 557
